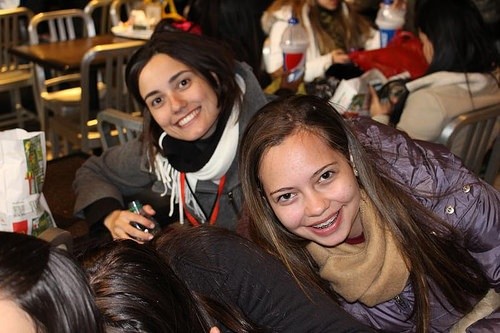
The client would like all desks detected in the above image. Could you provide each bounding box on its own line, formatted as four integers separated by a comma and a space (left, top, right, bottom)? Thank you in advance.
7, 36, 145, 114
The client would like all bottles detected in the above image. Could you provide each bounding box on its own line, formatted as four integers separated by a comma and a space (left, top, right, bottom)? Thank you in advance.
375, 0, 405, 48
129, 200, 161, 244
280, 17, 308, 94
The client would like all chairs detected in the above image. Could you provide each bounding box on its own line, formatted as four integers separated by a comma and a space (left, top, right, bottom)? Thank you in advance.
435, 102, 500, 182
0, 0, 176, 159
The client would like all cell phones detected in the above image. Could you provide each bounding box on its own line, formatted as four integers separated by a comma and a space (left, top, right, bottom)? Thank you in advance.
369, 79, 389, 103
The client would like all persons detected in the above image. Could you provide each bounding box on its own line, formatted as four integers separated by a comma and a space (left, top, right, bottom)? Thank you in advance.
151, 0, 261, 82
70, 29, 270, 244
81, 223, 380, 333
1, 231, 103, 333
369, 0, 499, 173
261, 0, 408, 83
236, 95, 500, 333
